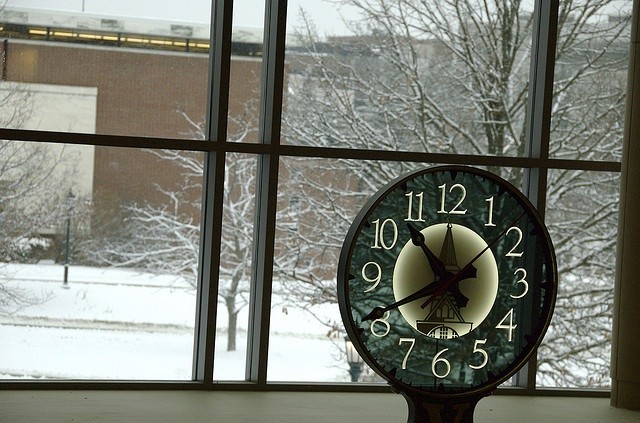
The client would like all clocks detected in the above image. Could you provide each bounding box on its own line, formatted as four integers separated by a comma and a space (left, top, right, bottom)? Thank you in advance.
336, 163, 561, 400
